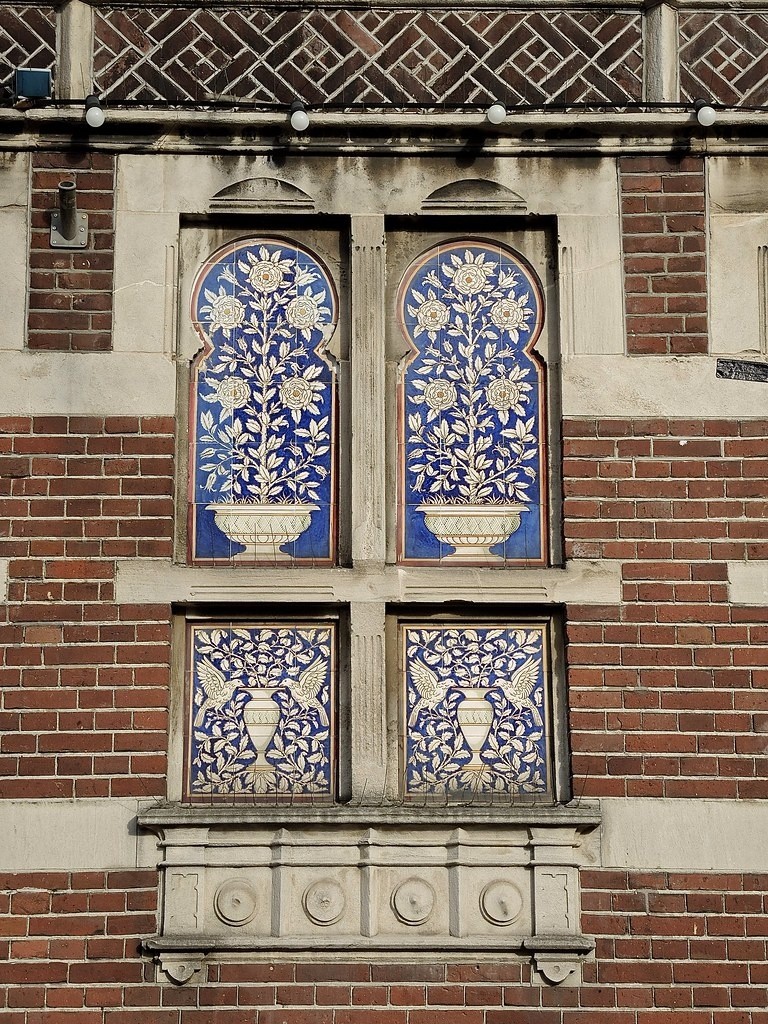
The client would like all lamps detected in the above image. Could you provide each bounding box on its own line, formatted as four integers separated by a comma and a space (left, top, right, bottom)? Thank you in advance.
14, 68, 51, 99
